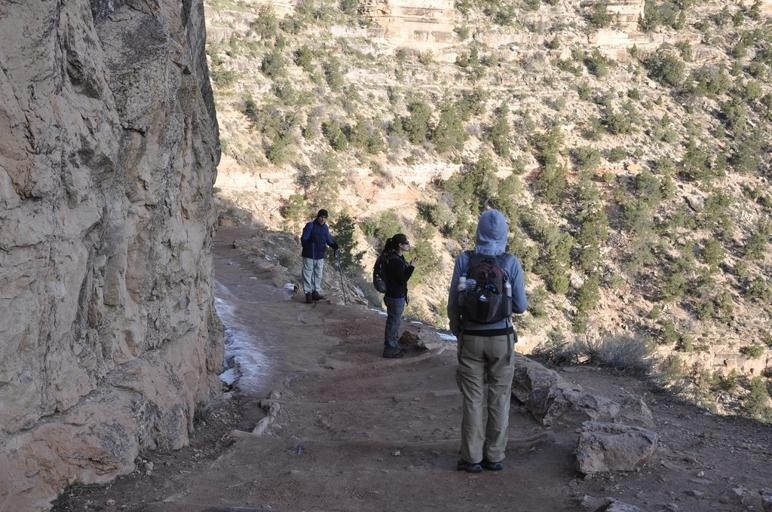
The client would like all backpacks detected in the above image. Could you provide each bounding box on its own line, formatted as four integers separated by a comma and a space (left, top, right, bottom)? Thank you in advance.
461, 250, 513, 324
374, 271, 389, 293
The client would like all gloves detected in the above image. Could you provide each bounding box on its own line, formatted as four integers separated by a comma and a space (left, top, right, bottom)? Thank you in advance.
332, 241, 338, 249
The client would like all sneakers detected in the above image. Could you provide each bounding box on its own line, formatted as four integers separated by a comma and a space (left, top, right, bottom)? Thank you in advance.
305, 293, 313, 303
383, 348, 409, 358
312, 291, 327, 299
483, 459, 502, 470
458, 458, 482, 472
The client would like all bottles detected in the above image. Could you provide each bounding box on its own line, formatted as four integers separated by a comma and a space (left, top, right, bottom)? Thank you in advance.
457, 275, 467, 306
503, 275, 513, 316
375, 273, 385, 292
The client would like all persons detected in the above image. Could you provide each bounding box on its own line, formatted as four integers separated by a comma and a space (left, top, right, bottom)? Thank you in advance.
447, 210, 528, 474
301, 208, 338, 303
375, 234, 419, 358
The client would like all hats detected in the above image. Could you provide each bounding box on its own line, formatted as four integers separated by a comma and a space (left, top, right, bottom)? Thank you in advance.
318, 209, 328, 218
